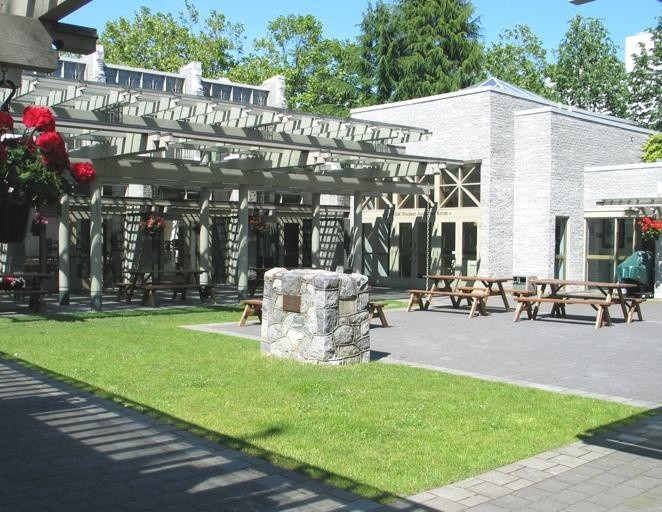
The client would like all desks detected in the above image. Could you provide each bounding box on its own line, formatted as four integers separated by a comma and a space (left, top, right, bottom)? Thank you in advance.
423, 274, 515, 317
128, 269, 205, 305
0, 272, 52, 313
247, 267, 321, 297
529, 279, 636, 327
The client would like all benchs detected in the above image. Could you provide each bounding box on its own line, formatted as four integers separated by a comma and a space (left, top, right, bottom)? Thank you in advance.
239, 299, 262, 326
112, 282, 214, 305
0, 289, 56, 313
367, 301, 388, 327
406, 287, 646, 329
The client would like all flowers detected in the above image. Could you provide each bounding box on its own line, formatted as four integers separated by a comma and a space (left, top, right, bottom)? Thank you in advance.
638, 215, 662, 240
29, 213, 48, 225
0, 105, 96, 211
139, 215, 165, 235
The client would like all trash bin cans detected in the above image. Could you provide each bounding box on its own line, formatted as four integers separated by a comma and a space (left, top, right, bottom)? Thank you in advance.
513, 275, 537, 296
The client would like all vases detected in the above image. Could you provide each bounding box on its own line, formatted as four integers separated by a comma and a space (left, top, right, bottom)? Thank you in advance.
1, 207, 30, 243
